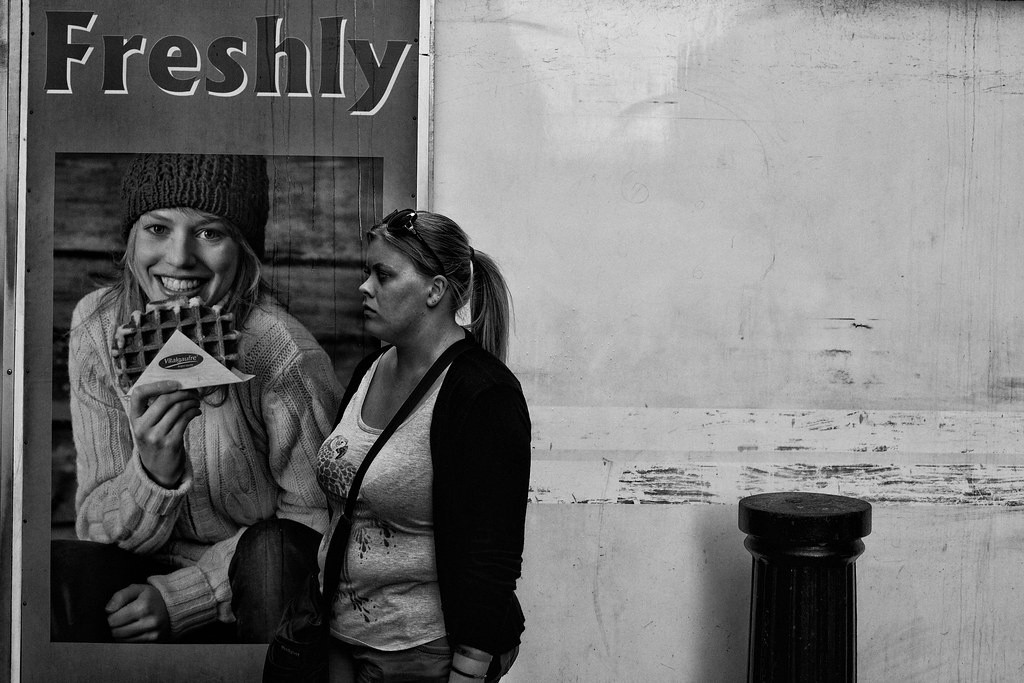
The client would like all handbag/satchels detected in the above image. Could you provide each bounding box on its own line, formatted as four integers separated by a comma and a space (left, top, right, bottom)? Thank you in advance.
261, 569, 334, 683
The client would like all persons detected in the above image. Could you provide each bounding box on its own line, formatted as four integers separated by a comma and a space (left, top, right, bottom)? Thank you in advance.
68, 153, 344, 644
316, 208, 530, 683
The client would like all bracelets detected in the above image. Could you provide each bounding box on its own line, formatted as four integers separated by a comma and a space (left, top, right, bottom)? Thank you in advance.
455, 647, 492, 662
451, 665, 487, 679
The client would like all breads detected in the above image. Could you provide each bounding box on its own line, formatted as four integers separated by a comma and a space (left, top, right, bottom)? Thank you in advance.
111, 297, 242, 389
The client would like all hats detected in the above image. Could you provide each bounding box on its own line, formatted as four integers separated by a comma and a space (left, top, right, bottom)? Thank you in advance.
119, 153, 269, 256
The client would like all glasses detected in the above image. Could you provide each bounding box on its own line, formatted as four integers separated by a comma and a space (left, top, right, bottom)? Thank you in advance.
380, 208, 446, 278
